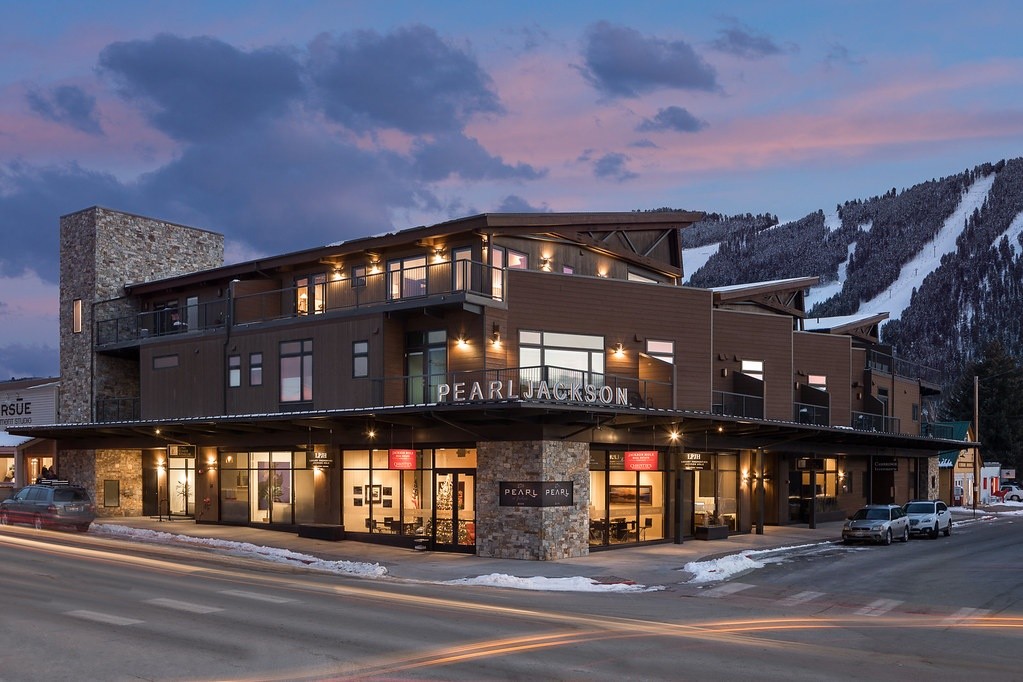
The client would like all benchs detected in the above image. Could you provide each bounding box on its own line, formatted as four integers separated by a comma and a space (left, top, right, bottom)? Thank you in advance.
297, 523, 345, 541
695, 524, 729, 541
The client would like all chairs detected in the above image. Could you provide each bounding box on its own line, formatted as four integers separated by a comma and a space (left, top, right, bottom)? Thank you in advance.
365, 516, 476, 545
589, 518, 646, 545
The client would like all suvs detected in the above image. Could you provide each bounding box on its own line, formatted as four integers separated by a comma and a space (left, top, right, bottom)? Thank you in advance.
901, 498, 953, 539
0, 480, 94, 533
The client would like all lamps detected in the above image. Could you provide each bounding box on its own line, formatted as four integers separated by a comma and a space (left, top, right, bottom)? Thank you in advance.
206, 458, 218, 473
367, 254, 384, 275
489, 332, 503, 350
454, 332, 470, 350
741, 470, 752, 486
154, 459, 166, 475
313, 464, 328, 480
613, 343, 625, 359
596, 271, 608, 278
431, 243, 448, 264
330, 262, 348, 281
838, 468, 847, 481
540, 254, 552, 272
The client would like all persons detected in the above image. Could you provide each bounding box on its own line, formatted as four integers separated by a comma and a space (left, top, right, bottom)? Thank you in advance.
42, 465, 48, 478
47, 470, 57, 480
3, 467, 14, 482
35, 474, 43, 484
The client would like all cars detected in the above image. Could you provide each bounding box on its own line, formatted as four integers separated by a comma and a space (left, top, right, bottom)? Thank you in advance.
842, 503, 911, 545
998, 485, 1023, 501
